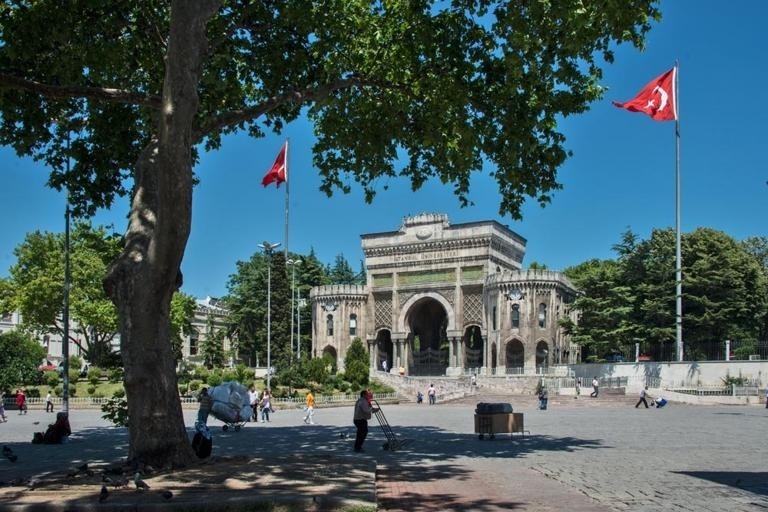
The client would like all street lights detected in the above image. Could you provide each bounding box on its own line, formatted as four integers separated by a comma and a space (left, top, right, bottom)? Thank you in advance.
257, 240, 282, 396
46, 112, 92, 437
287, 258, 303, 371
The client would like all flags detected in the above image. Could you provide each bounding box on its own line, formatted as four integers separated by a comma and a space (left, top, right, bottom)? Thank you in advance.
612, 67, 679, 122
261, 145, 287, 188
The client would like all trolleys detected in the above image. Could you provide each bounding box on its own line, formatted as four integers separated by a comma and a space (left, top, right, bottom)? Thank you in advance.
370, 400, 414, 452
478, 416, 495, 440
206, 405, 250, 433
650, 397, 667, 409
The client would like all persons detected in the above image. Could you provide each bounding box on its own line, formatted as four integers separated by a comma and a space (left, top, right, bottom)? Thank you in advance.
42, 412, 71, 445
247, 385, 275, 423
367, 388, 374, 406
303, 389, 316, 424
198, 387, 212, 424
591, 376, 599, 397
382, 360, 388, 372
428, 384, 436, 405
574, 381, 581, 399
191, 422, 212, 459
0, 388, 54, 423
539, 386, 548, 410
635, 386, 653, 408
353, 390, 372, 452
417, 391, 424, 404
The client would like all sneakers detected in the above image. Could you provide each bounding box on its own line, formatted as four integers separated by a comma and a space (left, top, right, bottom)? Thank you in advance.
353, 446, 366, 453
303, 418, 314, 425
252, 417, 270, 423
